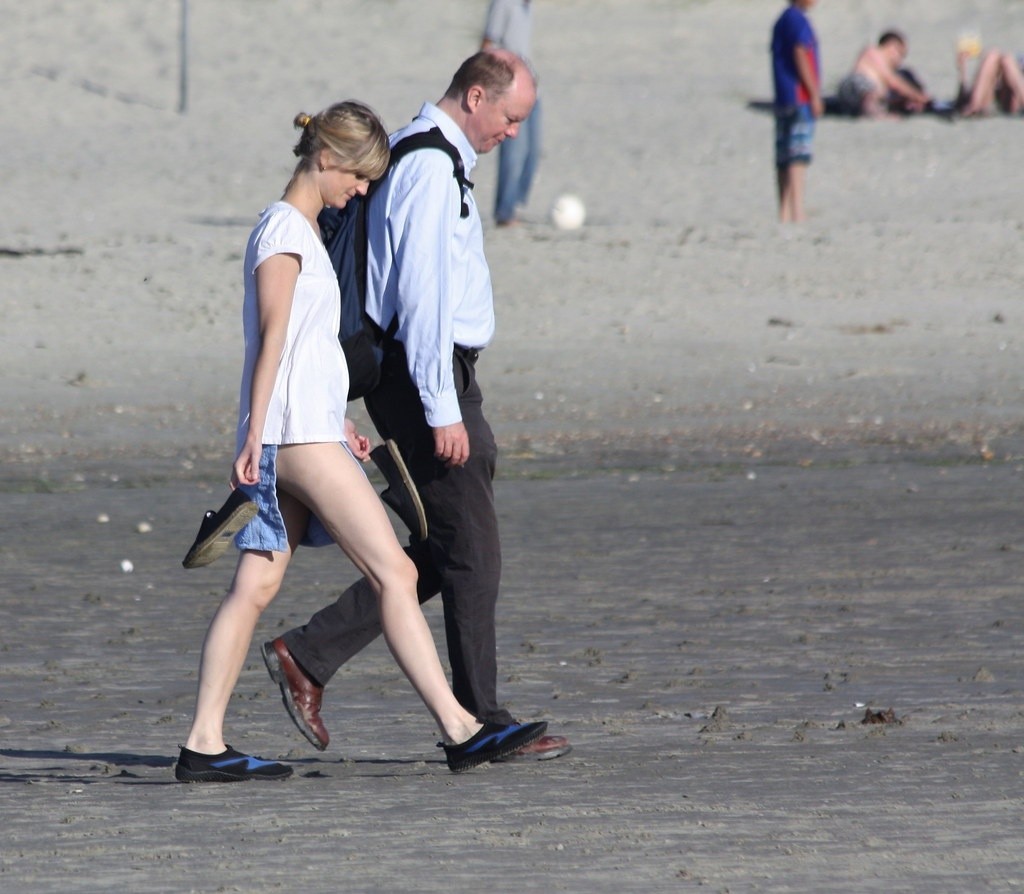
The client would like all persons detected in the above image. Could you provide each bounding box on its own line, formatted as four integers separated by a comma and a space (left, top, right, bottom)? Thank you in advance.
170, 97, 550, 785
839, 28, 1024, 125
769, 0, 826, 223
257, 44, 571, 768
478, 0, 545, 233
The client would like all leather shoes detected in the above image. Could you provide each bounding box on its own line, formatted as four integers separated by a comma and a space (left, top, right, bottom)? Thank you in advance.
261, 637, 329, 750
490, 735, 569, 761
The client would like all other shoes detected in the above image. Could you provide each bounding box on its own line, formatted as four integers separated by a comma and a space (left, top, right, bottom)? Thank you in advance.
174, 743, 294, 782
368, 439, 428, 541
437, 720, 548, 773
182, 486, 259, 569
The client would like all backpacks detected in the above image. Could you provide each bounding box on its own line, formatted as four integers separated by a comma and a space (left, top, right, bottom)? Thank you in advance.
317, 127, 474, 402
